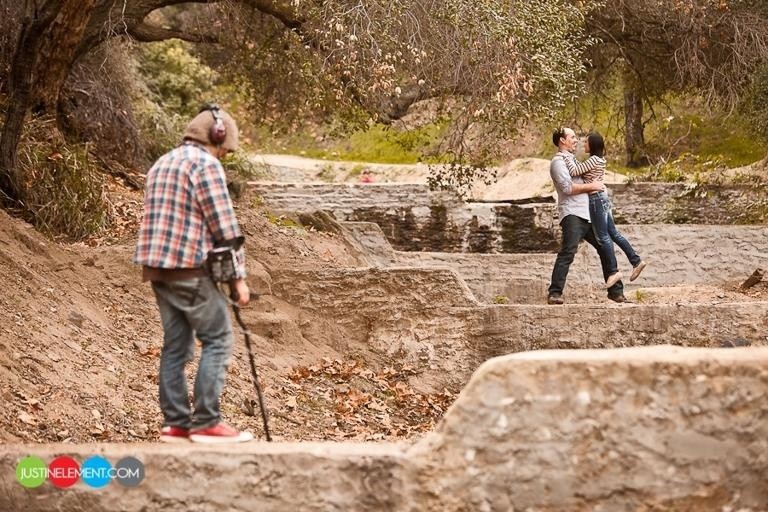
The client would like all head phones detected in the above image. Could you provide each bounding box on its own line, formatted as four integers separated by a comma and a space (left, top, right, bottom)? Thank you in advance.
199, 103, 227, 146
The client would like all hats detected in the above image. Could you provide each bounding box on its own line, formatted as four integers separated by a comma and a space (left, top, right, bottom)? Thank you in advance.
183, 108, 239, 152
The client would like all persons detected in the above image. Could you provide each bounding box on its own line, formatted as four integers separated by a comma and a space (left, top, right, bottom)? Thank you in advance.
560, 130, 647, 292
135, 107, 260, 444
545, 125, 633, 306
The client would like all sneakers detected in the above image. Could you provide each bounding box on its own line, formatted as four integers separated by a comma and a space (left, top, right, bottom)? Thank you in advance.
547, 292, 563, 305
607, 293, 634, 303
601, 270, 623, 289
160, 420, 253, 443
630, 261, 646, 282
